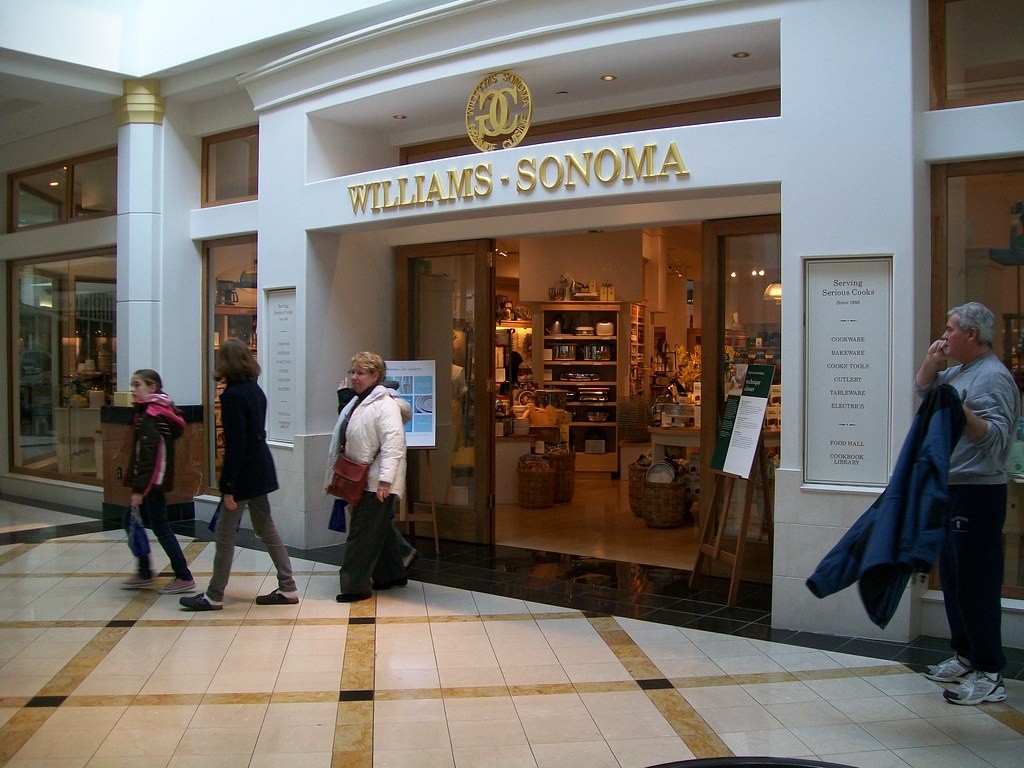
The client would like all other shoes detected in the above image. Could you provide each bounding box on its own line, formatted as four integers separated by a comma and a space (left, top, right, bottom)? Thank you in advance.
403, 548, 416, 567
372, 578, 408, 589
336, 591, 372, 602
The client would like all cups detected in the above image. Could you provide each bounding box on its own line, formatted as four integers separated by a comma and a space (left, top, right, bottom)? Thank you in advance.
85, 360, 95, 374
77, 363, 85, 373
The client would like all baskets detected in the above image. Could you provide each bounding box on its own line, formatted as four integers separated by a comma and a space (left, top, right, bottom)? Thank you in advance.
628, 462, 686, 529
518, 445, 575, 509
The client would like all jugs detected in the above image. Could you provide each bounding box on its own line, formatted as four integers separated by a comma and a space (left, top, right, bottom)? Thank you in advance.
545, 319, 561, 334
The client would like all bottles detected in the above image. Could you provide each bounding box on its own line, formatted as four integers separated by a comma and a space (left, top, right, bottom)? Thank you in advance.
89, 386, 105, 408
230, 326, 236, 337
242, 331, 250, 345
653, 352, 671, 372
600, 284, 616, 301
684, 466, 701, 525
662, 343, 669, 353
252, 333, 257, 348
520, 368, 533, 380
225, 287, 239, 305
98, 343, 112, 373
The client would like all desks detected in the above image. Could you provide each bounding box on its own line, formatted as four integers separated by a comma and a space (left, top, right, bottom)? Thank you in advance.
646, 425, 780, 466
52, 406, 103, 481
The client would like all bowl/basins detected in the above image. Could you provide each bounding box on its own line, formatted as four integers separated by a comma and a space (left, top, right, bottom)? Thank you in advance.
513, 406, 528, 418
645, 461, 675, 484
547, 287, 566, 302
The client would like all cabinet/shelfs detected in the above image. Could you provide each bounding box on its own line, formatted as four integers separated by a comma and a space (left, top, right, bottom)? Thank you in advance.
494, 300, 647, 505
651, 326, 781, 404
461, 327, 475, 444
214, 304, 258, 378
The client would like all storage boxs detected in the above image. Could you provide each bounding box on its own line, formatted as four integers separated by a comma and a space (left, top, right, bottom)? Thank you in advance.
689, 453, 700, 475
764, 385, 782, 428
214, 332, 219, 346
234, 288, 257, 306
684, 473, 701, 499
585, 439, 606, 454
597, 322, 614, 335
214, 382, 227, 458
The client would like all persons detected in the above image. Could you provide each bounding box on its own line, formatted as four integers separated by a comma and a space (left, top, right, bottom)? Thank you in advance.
911, 301, 1021, 706
119, 368, 197, 594
452, 363, 468, 453
323, 351, 419, 603
179, 337, 301, 610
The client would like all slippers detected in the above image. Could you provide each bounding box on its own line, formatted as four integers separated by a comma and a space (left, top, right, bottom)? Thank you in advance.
256, 588, 300, 605
179, 593, 224, 611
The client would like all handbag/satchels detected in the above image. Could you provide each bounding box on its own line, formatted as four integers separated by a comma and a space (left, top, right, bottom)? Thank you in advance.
128, 503, 151, 557
327, 453, 371, 507
327, 498, 354, 536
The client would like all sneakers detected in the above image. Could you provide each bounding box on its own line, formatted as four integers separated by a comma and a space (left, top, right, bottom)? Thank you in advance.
119, 576, 159, 589
942, 669, 1008, 705
924, 651, 976, 684
157, 577, 196, 594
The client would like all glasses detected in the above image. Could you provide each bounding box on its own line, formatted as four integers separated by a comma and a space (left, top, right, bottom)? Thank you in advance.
347, 370, 365, 375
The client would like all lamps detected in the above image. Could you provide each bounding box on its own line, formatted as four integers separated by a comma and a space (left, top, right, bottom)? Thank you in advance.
500, 248, 508, 257
762, 233, 782, 301
